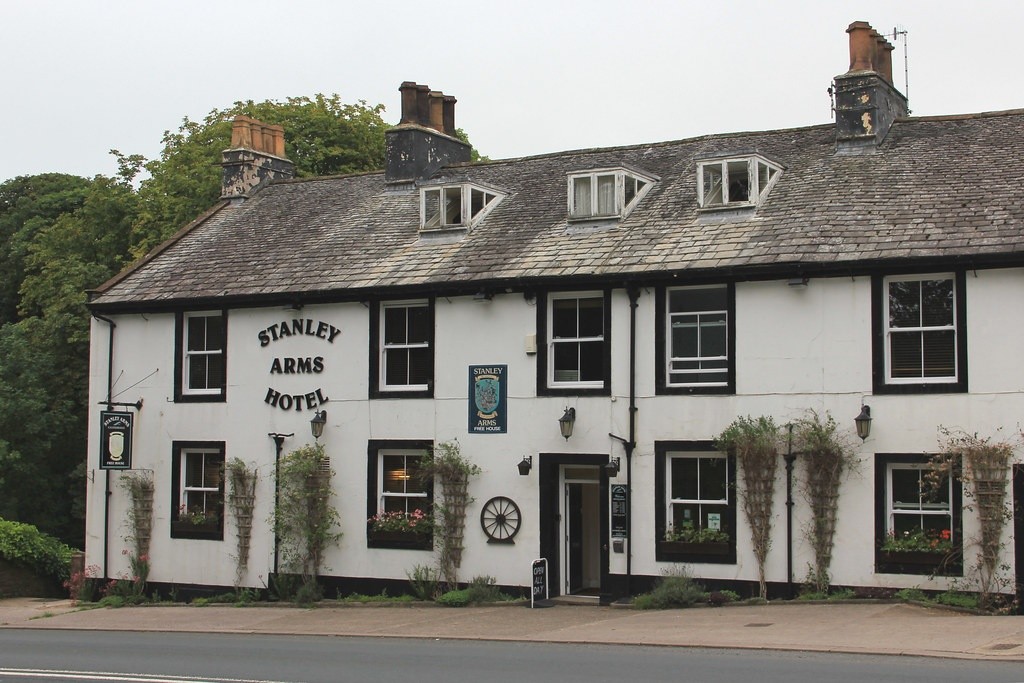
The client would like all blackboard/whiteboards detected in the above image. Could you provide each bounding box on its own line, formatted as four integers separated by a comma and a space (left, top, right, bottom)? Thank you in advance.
531, 558, 549, 602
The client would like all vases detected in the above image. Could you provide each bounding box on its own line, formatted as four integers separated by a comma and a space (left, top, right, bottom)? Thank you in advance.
883, 550, 952, 566
174, 522, 218, 535
370, 529, 429, 544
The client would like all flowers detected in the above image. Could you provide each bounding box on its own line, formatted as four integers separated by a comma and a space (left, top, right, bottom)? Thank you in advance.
879, 527, 955, 553
176, 504, 218, 525
365, 507, 432, 532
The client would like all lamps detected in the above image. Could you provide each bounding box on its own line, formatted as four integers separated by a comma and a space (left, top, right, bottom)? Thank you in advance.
603, 456, 621, 478
853, 405, 873, 443
473, 287, 494, 305
558, 406, 576, 442
310, 409, 327, 440
517, 455, 533, 475
786, 276, 810, 289
283, 300, 304, 312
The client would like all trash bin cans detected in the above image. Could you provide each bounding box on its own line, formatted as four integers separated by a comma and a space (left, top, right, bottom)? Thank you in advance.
69, 551, 98, 601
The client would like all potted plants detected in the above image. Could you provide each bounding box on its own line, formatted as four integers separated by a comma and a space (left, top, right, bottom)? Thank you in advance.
658, 523, 730, 556
